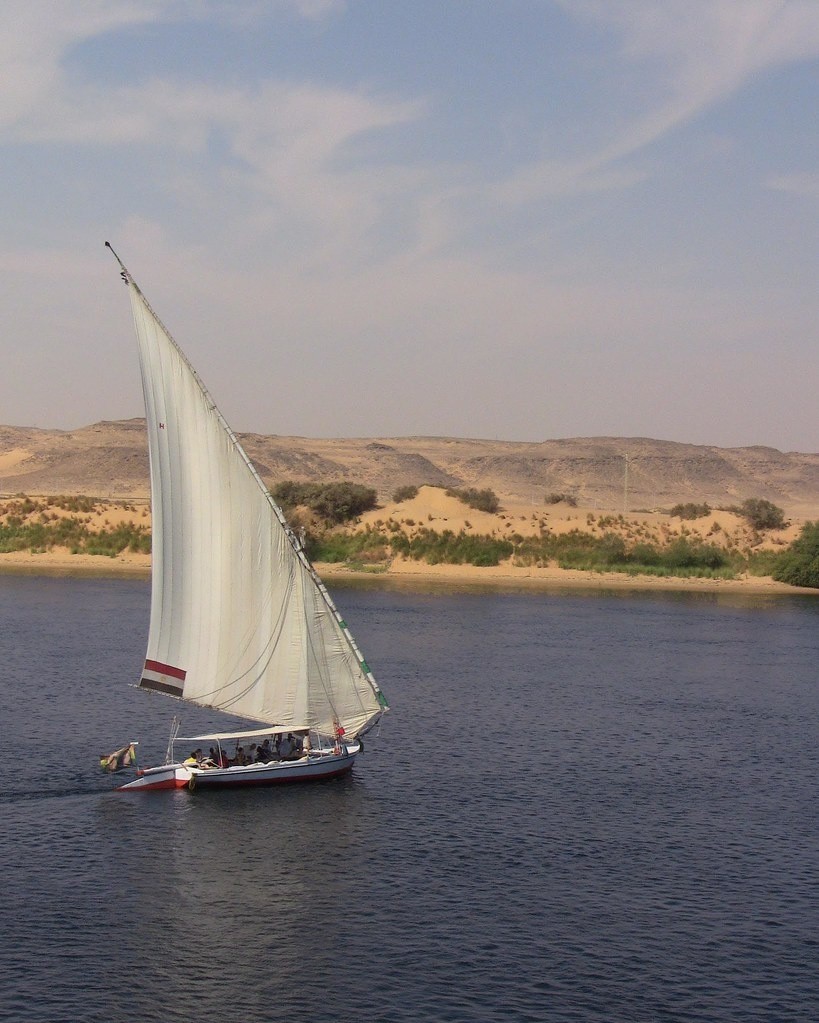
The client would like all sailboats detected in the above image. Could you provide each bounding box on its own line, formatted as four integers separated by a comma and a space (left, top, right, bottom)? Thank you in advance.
105, 240, 387, 790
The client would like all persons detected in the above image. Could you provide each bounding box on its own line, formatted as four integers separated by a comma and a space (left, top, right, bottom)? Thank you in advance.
288, 733, 295, 742
303, 732, 312, 750
210, 748, 218, 765
257, 739, 275, 763
278, 738, 290, 756
250, 744, 257, 764
216, 747, 232, 768
184, 752, 198, 763
296, 736, 301, 747
235, 747, 245, 766
276, 734, 282, 755
195, 749, 205, 763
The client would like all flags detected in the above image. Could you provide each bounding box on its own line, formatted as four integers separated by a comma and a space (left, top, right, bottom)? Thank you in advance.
99, 745, 136, 774
